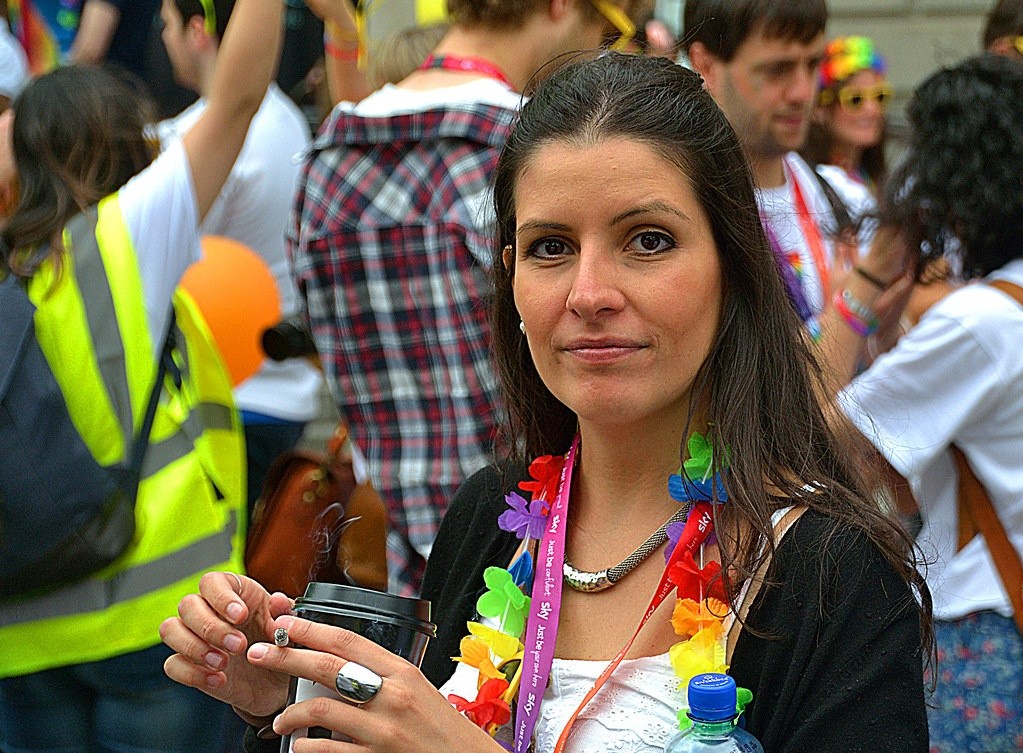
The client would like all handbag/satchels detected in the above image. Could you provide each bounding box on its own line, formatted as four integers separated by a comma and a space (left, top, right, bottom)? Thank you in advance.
243, 447, 355, 605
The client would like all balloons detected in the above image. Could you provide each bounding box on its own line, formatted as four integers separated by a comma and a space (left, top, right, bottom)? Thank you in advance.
181, 235, 280, 391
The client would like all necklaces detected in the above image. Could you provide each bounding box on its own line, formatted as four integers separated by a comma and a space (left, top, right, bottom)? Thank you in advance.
562, 502, 696, 592
448, 433, 751, 738
417, 53, 517, 93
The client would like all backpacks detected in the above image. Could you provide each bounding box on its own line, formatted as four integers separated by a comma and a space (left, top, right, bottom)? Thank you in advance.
0, 232, 177, 602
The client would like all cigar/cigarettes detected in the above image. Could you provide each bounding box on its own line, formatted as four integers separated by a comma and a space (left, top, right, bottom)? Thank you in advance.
274, 628, 290, 648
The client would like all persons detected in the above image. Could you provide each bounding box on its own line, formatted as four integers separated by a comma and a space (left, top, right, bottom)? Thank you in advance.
161, 0, 1023, 753
0, 0, 282, 753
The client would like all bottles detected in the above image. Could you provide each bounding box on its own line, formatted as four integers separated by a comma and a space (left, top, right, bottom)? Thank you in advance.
663, 673, 763, 753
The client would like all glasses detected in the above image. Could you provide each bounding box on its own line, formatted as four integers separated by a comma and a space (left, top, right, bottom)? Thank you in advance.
591, 0, 637, 53
817, 87, 893, 116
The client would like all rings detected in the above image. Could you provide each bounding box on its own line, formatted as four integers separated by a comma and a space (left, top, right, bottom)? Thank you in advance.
224, 572, 241, 595
335, 662, 383, 708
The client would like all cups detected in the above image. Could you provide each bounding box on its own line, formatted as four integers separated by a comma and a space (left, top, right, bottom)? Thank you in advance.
279, 581, 437, 753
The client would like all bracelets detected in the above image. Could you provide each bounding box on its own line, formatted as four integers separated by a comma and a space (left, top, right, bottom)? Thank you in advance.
842, 287, 881, 327
854, 265, 889, 293
324, 30, 358, 63
831, 287, 875, 338
